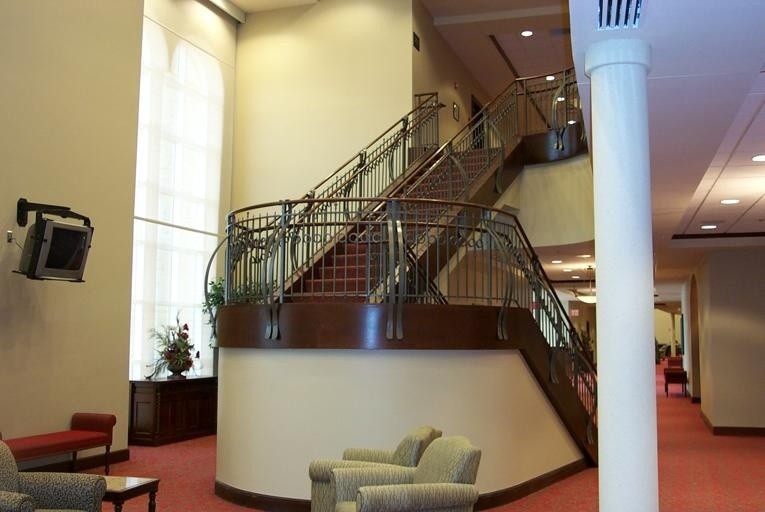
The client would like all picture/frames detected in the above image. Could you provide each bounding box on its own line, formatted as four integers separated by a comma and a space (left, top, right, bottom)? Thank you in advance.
453, 102, 459, 121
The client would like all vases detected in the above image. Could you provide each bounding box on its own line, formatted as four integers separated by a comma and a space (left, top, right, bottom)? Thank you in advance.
167, 363, 186, 379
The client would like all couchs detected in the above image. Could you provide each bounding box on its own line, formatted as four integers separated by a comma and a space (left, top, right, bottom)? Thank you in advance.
309, 424, 442, 511
329, 435, 482, 511
0, 442, 107, 511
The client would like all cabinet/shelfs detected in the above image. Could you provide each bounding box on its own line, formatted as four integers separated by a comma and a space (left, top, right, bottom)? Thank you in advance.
129, 376, 217, 447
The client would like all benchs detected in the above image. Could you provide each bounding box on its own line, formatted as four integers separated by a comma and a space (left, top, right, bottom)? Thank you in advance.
668, 356, 682, 367
0, 413, 117, 474
664, 368, 687, 397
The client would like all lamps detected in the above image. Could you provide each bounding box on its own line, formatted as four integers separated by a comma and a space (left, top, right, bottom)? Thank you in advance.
567, 265, 596, 304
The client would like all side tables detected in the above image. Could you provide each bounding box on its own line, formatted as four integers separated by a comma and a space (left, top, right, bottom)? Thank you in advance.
99, 476, 161, 511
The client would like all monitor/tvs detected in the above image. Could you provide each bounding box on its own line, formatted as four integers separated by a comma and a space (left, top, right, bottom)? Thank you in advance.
18, 216, 93, 280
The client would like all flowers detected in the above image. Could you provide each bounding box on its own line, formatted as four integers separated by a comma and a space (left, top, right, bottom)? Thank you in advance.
146, 325, 200, 372
